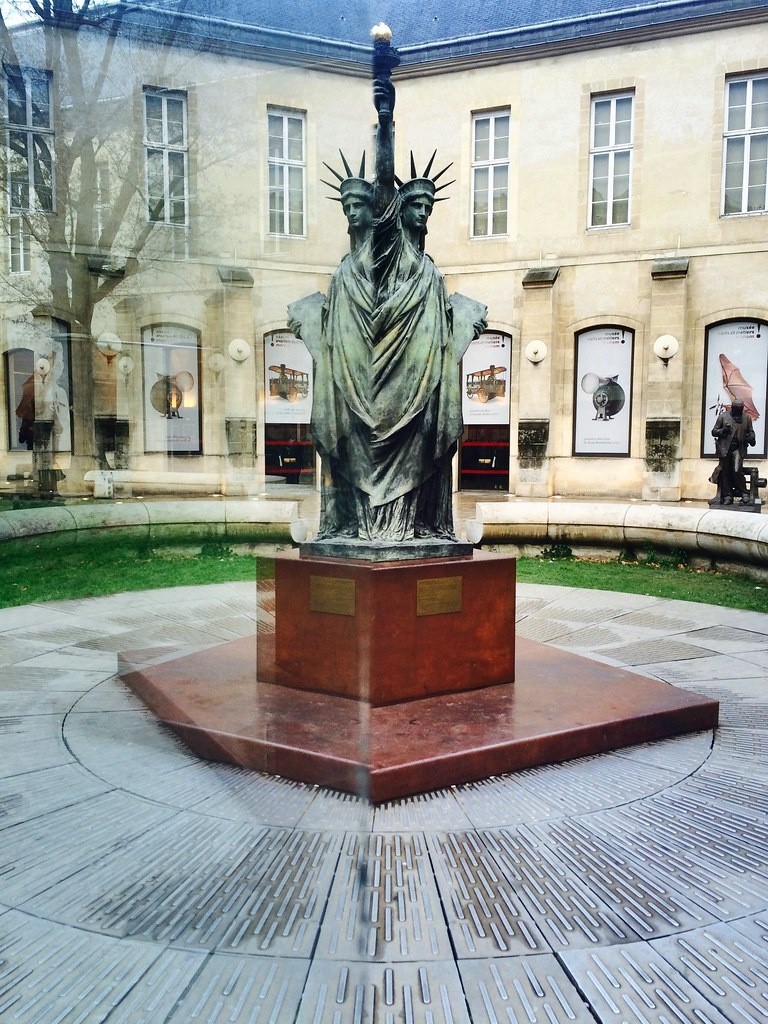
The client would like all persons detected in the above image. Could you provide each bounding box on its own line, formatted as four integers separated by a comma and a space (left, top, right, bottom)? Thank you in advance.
374, 24, 489, 537
284, 147, 373, 540
711, 400, 755, 506
285, 437, 304, 482
